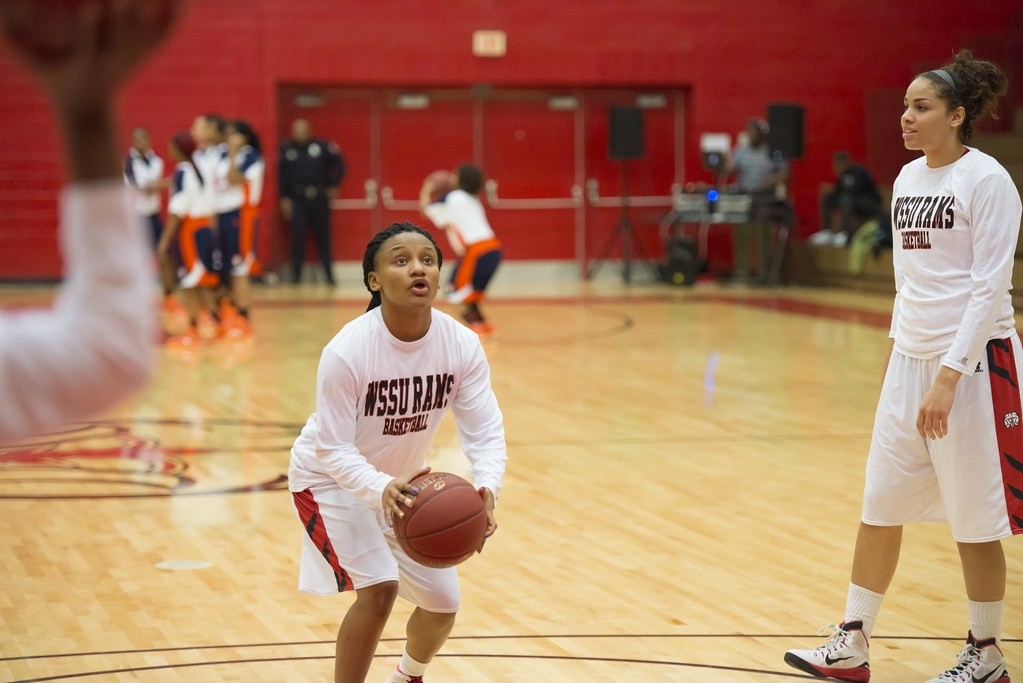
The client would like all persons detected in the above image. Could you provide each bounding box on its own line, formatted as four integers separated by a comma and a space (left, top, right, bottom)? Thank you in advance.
419, 164, 503, 331
125, 126, 163, 238
157, 114, 266, 332
716, 120, 787, 286
806, 153, 879, 248
287, 223, 508, 683
278, 118, 345, 286
0, 1, 182, 431
781, 49, 1023, 683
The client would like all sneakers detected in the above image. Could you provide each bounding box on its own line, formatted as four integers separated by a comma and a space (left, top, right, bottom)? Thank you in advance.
784, 624, 871, 683
926, 644, 1010, 683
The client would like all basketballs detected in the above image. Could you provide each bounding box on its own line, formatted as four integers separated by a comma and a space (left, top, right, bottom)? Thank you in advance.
5, 0, 174, 55
392, 472, 487, 569
424, 169, 458, 203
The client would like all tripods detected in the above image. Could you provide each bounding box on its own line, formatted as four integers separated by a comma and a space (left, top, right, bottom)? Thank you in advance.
586, 158, 659, 289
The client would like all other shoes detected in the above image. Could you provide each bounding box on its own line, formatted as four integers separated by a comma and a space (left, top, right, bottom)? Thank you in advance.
153, 298, 252, 349
468, 318, 492, 335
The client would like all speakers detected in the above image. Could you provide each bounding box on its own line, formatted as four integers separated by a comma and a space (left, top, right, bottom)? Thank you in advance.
765, 103, 805, 160
608, 107, 643, 160
